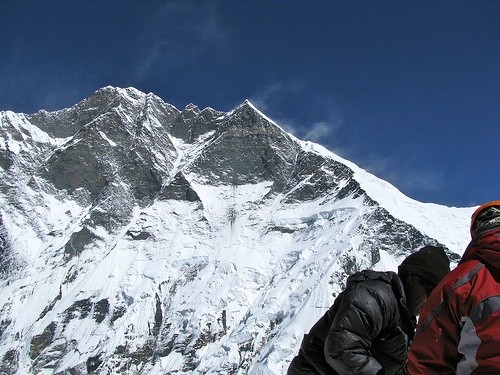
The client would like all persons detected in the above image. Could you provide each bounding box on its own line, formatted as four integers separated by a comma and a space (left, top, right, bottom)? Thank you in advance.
287, 245, 450, 375
405, 201, 500, 375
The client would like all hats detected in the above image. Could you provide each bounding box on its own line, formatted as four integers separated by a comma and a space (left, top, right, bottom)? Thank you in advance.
469, 200, 500, 237
398, 244, 450, 282
